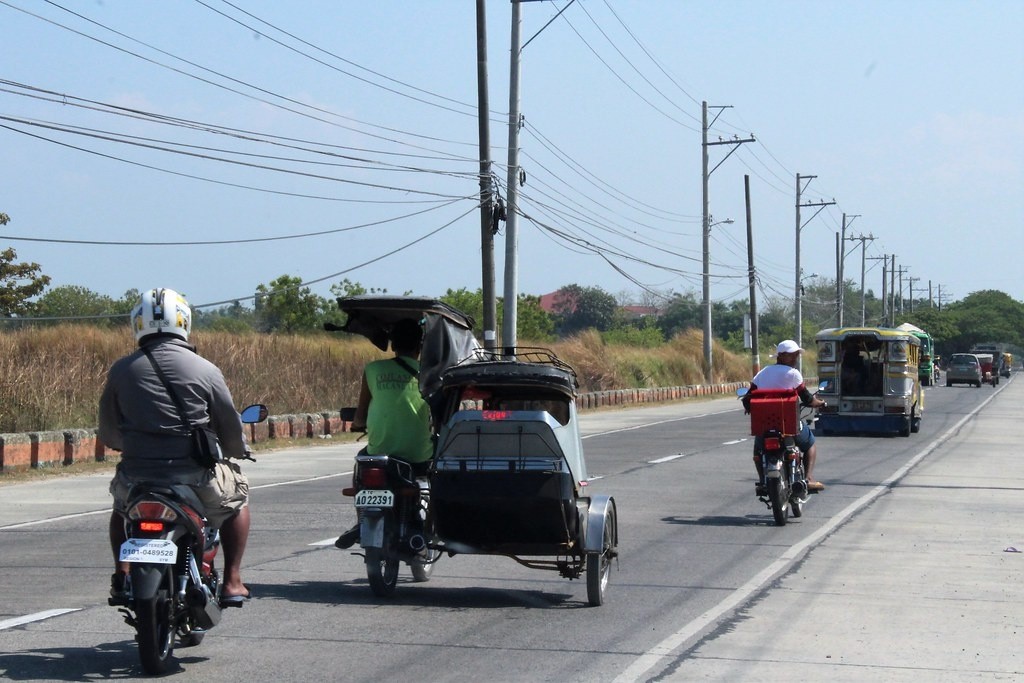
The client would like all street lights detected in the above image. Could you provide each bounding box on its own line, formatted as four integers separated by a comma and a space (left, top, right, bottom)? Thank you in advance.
795, 274, 819, 376
702, 219, 734, 384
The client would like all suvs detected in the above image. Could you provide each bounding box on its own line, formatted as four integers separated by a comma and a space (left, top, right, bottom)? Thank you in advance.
972, 344, 1012, 388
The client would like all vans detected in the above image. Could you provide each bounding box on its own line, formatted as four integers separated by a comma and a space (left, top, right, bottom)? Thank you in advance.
946, 353, 981, 387
814, 327, 924, 437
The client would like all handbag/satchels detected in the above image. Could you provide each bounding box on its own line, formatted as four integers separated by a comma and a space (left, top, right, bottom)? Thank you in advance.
193, 427, 223, 468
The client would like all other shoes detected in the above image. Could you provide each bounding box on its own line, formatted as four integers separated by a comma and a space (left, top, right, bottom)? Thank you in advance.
335, 524, 359, 549
807, 477, 825, 491
756, 484, 767, 494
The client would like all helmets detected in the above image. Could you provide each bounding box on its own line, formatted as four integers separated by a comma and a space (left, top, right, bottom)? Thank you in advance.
130, 288, 191, 345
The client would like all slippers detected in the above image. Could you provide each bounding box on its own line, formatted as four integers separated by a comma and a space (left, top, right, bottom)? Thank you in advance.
222, 584, 252, 603
109, 589, 132, 599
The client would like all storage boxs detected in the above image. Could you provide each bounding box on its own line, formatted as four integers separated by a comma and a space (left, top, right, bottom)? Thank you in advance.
750, 389, 801, 436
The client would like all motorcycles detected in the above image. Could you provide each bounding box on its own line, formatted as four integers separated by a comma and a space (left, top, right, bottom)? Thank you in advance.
323, 296, 621, 606
735, 381, 833, 524
107, 404, 268, 674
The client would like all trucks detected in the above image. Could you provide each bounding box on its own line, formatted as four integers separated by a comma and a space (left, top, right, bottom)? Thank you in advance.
895, 323, 934, 386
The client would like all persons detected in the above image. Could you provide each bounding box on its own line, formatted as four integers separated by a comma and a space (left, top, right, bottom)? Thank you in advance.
745, 339, 826, 492
334, 319, 439, 548
97, 287, 252, 606
842, 347, 871, 392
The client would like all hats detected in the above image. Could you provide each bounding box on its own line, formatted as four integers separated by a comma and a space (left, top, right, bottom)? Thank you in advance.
774, 340, 805, 355
